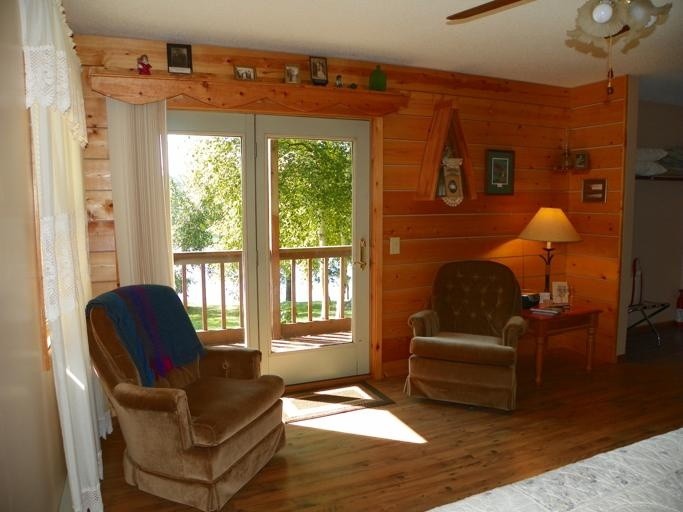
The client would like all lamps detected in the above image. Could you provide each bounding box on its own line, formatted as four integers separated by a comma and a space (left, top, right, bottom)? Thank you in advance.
84, 284, 286, 512
403, 259, 527, 412
626, 257, 672, 348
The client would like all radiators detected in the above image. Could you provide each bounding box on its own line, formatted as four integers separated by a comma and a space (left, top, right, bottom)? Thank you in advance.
530, 307, 562, 314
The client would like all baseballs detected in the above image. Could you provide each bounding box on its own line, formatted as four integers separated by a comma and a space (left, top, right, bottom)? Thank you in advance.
675, 288, 683, 325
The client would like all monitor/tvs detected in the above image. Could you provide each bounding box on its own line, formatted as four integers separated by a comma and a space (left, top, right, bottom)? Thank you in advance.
528, 299, 571, 318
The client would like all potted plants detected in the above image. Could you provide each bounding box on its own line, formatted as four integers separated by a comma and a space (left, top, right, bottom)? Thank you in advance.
309, 56, 328, 86
485, 149, 514, 195
166, 43, 193, 75
283, 64, 301, 84
236, 65, 256, 82
574, 151, 587, 169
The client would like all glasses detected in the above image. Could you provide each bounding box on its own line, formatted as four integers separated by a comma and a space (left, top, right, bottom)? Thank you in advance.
564, 0, 672, 94
517, 207, 584, 299
